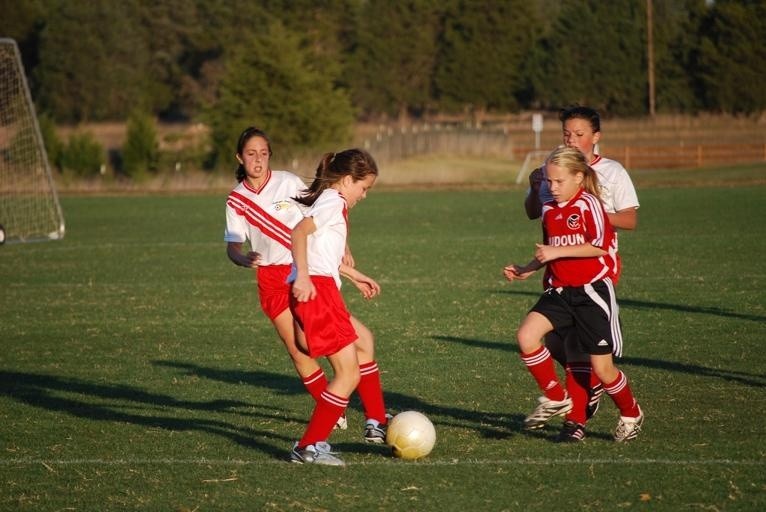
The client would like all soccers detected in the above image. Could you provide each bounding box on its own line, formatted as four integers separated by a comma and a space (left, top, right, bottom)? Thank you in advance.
387, 410, 438, 460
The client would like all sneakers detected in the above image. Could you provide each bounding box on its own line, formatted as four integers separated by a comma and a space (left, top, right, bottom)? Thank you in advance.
364, 422, 389, 444
551, 420, 589, 446
584, 384, 606, 419
287, 441, 346, 469
521, 388, 577, 430
331, 415, 349, 430
611, 403, 645, 444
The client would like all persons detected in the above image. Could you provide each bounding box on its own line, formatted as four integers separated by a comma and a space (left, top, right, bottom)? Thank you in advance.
523, 105, 642, 419
502, 143, 646, 443
286, 149, 396, 469
222, 124, 356, 432
